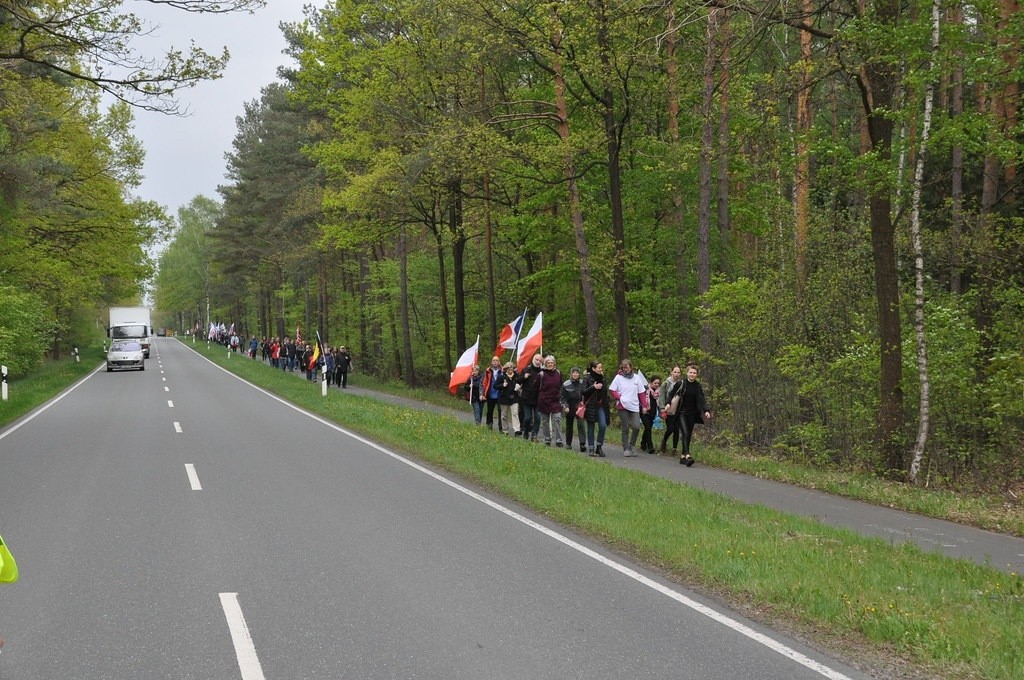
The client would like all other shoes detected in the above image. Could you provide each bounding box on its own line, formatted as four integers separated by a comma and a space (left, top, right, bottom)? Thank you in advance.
589, 449, 596, 456
566, 443, 572, 449
686, 457, 694, 466
556, 442, 564, 447
515, 431, 522, 437
596, 449, 605, 457
546, 443, 553, 447
524, 432, 529, 440
532, 438, 539, 443
660, 439, 666, 453
649, 448, 656, 454
580, 445, 587, 452
624, 449, 630, 456
680, 457, 687, 465
672, 450, 679, 457
630, 445, 638, 457
641, 441, 647, 450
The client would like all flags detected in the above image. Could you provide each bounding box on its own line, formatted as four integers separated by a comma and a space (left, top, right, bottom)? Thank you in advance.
195, 323, 237, 337
496, 308, 527, 356
296, 321, 303, 345
449, 338, 480, 395
515, 312, 542, 372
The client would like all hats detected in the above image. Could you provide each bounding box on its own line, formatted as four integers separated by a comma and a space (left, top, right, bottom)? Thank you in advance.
570, 367, 581, 375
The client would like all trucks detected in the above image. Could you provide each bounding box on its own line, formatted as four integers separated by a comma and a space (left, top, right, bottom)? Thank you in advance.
107, 307, 154, 360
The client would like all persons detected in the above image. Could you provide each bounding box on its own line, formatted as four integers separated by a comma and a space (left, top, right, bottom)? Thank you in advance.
665, 366, 710, 466
494, 361, 521, 437
518, 354, 545, 443
536, 355, 564, 449
559, 366, 588, 451
639, 375, 662, 455
481, 355, 503, 434
463, 363, 482, 425
193, 329, 352, 389
580, 360, 616, 459
658, 366, 683, 457
607, 359, 649, 457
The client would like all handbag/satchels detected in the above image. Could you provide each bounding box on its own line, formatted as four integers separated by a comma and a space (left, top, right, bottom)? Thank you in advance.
575, 402, 587, 418
518, 389, 522, 397
666, 381, 683, 415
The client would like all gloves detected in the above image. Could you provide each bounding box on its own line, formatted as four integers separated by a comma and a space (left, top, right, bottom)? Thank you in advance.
660, 411, 667, 420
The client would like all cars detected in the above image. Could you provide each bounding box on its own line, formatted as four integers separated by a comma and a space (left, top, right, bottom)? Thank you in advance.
103, 340, 147, 372
156, 328, 167, 337
